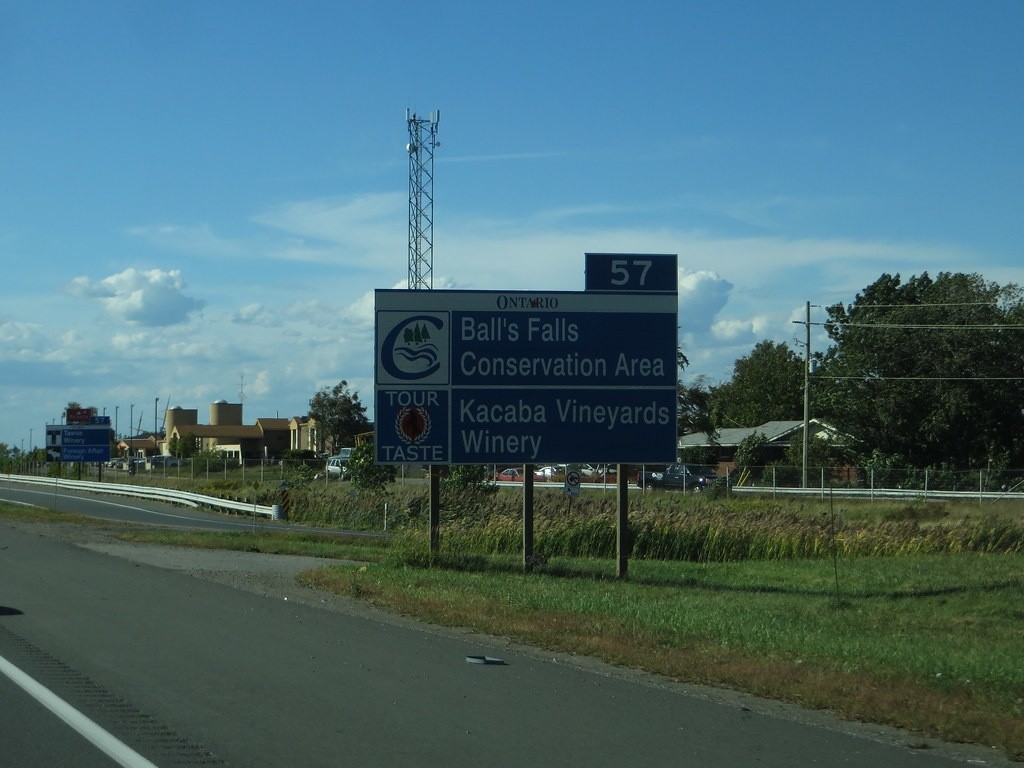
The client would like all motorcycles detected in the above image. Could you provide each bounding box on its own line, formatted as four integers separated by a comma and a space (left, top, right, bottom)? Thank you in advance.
128, 468, 136, 477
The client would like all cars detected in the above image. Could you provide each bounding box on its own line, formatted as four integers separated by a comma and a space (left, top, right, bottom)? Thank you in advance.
100, 456, 184, 471
325, 447, 357, 480
495, 463, 605, 482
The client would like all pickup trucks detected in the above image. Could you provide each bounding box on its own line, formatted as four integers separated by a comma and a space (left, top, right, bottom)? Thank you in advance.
635, 463, 730, 494
